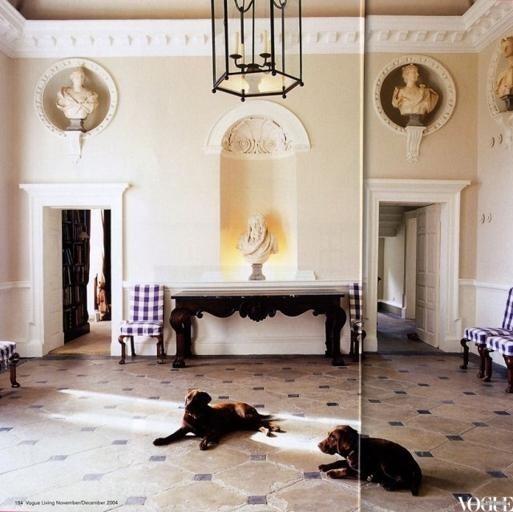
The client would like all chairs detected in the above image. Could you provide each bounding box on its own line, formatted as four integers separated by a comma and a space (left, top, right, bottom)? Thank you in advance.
117, 284, 167, 364
459, 287, 513, 392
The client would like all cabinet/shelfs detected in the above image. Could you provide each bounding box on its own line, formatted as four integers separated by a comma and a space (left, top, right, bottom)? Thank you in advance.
62, 210, 90, 343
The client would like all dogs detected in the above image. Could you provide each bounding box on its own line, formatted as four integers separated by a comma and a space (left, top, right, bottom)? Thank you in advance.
318, 423, 423, 497
152, 388, 281, 450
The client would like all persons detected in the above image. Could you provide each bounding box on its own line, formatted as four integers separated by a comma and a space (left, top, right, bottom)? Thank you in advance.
54, 69, 100, 119
391, 64, 440, 115
492, 34, 513, 97
234, 210, 278, 263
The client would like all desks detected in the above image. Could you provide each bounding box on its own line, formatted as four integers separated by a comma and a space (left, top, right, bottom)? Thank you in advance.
169, 286, 345, 367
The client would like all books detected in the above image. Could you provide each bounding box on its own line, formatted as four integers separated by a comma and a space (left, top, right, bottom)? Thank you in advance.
61, 209, 91, 329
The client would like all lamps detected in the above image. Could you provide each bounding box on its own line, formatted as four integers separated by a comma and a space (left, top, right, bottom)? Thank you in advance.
208, 1, 304, 100
